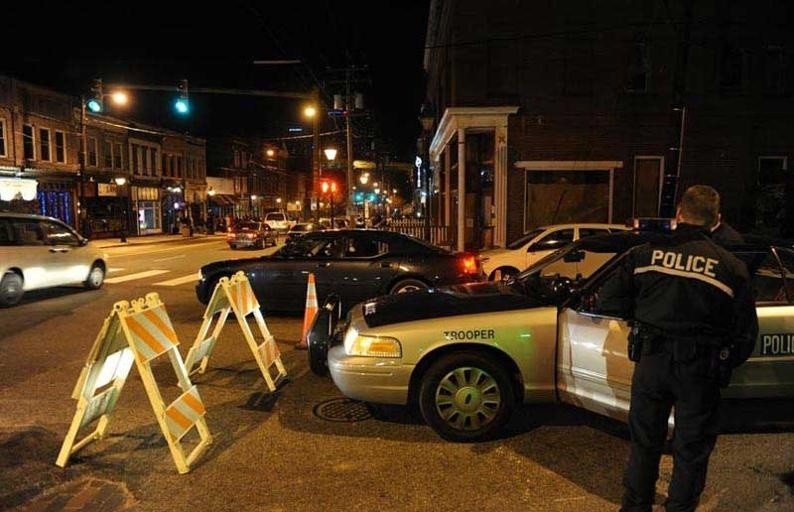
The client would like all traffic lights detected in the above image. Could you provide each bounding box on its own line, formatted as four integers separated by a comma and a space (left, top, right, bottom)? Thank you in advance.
176, 79, 189, 113
320, 178, 336, 195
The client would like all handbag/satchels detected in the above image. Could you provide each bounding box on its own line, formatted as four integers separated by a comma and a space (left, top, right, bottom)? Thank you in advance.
628, 320, 648, 362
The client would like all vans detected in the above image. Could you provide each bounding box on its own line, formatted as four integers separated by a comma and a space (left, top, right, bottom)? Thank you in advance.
0, 211, 108, 307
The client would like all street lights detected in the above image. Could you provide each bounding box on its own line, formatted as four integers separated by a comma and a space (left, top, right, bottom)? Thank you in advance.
324, 148, 337, 228
115, 177, 126, 243
81, 96, 101, 237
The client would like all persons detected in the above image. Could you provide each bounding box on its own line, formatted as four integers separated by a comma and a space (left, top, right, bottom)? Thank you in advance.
597, 185, 759, 512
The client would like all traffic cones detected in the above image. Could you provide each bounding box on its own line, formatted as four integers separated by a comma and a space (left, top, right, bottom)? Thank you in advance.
295, 272, 320, 350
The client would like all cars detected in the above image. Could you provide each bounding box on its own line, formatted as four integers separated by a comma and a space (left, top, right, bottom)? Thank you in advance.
478, 222, 636, 283
195, 228, 486, 318
225, 221, 276, 248
317, 215, 379, 228
284, 223, 327, 244
324, 228, 794, 444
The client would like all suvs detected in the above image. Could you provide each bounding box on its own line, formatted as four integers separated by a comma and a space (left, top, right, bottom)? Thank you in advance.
263, 211, 290, 233
